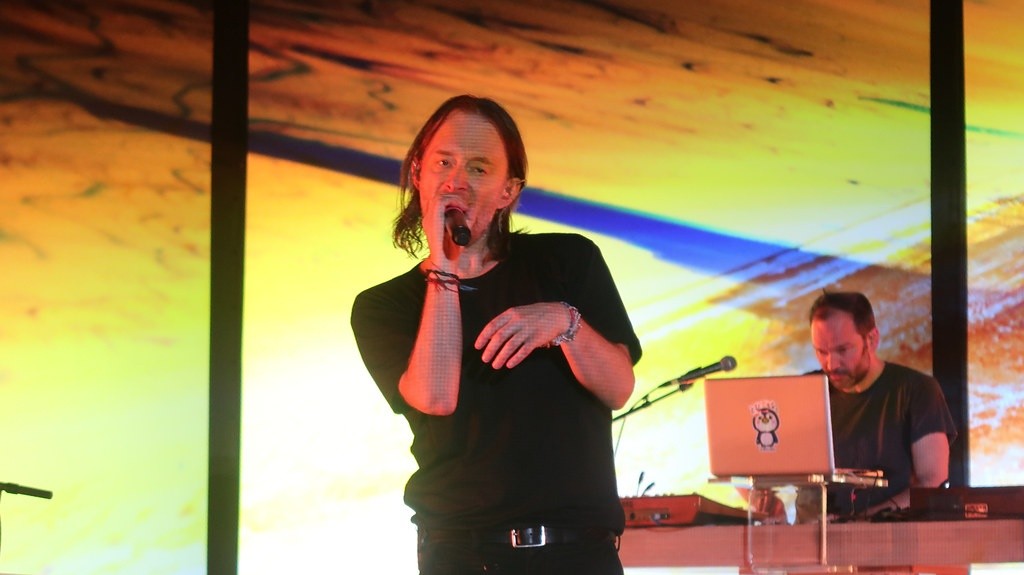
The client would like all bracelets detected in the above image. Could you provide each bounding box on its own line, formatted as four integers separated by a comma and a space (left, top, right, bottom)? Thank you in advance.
890, 498, 899, 508
552, 302, 581, 346
423, 269, 474, 293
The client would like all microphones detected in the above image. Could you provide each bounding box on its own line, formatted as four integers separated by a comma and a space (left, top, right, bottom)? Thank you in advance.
443, 205, 471, 246
659, 356, 737, 389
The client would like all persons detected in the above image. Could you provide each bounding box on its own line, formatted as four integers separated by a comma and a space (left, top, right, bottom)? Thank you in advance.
802, 290, 958, 515
350, 94, 643, 575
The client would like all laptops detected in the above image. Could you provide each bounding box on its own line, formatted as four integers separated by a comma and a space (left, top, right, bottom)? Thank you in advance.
705, 374, 884, 477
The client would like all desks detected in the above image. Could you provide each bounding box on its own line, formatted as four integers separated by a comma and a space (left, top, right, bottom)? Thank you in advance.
616, 520, 1024, 575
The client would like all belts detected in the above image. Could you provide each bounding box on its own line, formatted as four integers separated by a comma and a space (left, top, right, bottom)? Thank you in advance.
417, 524, 617, 549
